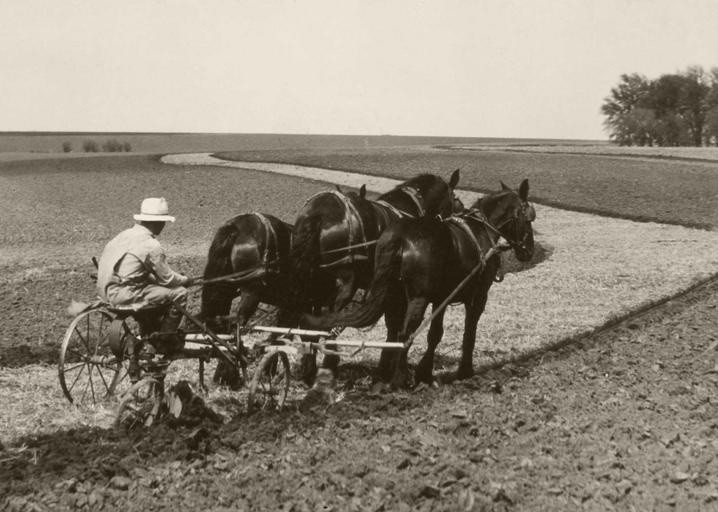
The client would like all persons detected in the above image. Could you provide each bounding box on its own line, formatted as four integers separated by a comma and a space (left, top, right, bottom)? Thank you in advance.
97, 197, 193, 350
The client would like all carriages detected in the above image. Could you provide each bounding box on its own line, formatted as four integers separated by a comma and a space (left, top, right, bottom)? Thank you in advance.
55, 165, 545, 432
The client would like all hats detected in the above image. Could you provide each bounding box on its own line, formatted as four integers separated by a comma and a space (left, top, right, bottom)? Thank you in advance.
133, 197, 176, 222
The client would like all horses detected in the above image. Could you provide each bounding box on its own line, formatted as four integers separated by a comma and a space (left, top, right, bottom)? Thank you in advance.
317, 179, 537, 390
196, 184, 370, 392
262, 169, 465, 388
